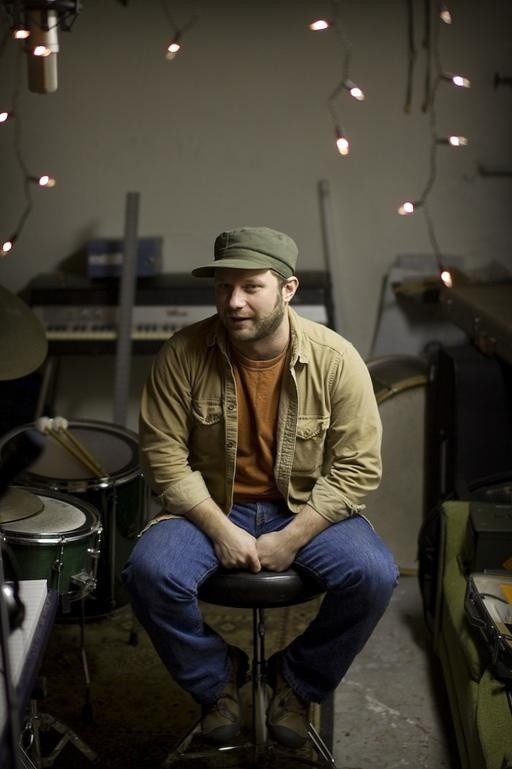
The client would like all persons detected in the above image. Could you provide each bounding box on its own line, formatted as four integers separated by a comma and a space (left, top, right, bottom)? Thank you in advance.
119, 223, 401, 750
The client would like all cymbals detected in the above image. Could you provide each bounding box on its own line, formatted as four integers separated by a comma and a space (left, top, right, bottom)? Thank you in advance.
2, 285, 49, 382
1, 486, 45, 522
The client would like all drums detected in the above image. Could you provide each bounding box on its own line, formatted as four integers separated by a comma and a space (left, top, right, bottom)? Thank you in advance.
3, 494, 104, 609
362, 353, 424, 578
1, 417, 147, 615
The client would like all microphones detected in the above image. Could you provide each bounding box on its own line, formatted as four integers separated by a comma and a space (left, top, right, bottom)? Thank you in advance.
26, 9, 59, 95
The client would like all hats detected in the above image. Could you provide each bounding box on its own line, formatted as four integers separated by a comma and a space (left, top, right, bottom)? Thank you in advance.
193, 227, 299, 280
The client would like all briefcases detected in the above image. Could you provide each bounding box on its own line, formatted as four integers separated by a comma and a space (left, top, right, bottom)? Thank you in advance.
465, 571, 512, 682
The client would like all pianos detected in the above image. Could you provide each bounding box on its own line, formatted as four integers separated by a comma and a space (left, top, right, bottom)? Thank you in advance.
2, 580, 60, 743
21, 271, 335, 355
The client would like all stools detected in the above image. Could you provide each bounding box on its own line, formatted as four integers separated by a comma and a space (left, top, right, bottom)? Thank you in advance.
165, 560, 340, 769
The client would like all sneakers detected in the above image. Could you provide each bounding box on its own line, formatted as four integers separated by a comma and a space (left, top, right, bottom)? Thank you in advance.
262, 660, 309, 748
200, 646, 248, 740
